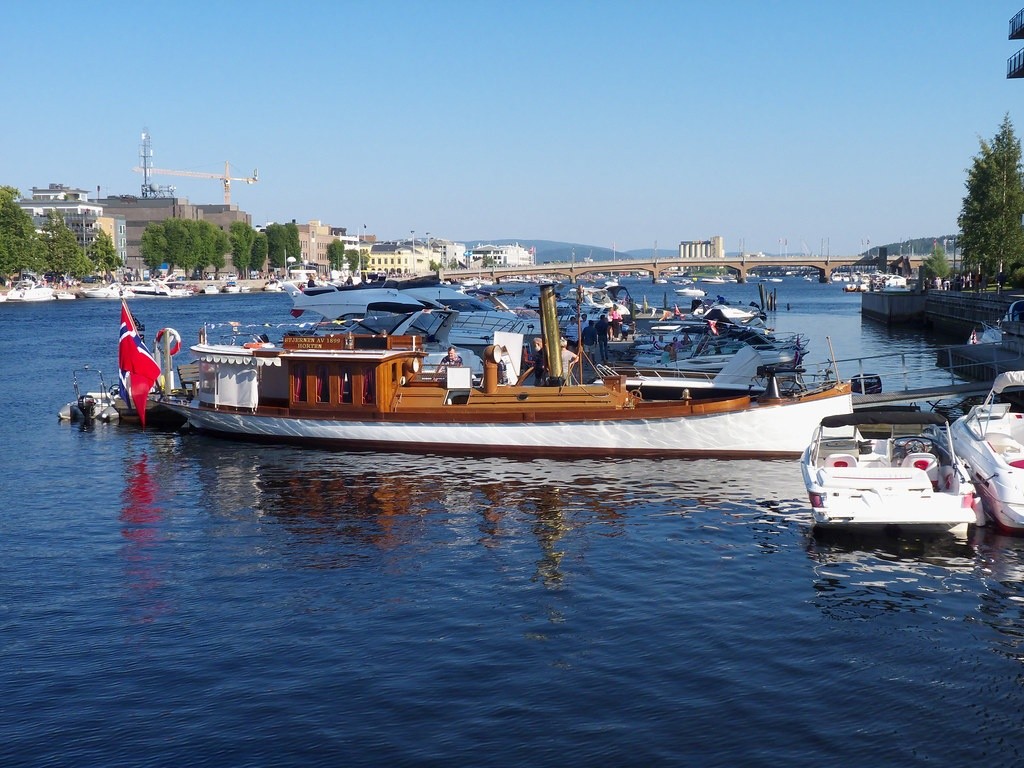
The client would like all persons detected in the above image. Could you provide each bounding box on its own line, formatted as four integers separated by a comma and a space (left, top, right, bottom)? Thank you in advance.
97, 274, 129, 288
432, 347, 463, 381
37, 273, 81, 289
531, 304, 623, 387
934, 274, 970, 291
657, 335, 692, 365
5, 278, 12, 289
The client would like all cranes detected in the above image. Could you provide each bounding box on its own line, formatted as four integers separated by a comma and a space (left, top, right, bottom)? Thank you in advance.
140, 167, 258, 205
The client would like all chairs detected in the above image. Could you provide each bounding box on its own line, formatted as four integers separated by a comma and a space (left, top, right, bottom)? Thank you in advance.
901, 452, 940, 487
824, 453, 858, 468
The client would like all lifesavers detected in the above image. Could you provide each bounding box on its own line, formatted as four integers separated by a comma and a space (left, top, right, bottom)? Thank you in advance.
244, 342, 275, 349
154, 327, 182, 357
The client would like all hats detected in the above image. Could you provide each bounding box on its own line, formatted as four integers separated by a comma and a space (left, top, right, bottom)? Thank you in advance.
560, 336, 567, 347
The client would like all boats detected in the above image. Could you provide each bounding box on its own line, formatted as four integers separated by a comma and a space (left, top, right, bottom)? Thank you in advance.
6, 266, 878, 461
800, 410, 987, 535
949, 371, 1024, 535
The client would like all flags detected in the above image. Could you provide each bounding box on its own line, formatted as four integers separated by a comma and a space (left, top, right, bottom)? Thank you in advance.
118, 300, 162, 428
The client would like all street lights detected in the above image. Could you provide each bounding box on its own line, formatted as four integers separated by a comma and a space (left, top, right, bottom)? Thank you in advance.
426, 232, 431, 272
411, 230, 417, 272
358, 224, 367, 279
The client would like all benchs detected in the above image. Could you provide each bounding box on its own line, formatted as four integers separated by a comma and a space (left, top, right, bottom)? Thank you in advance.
177, 365, 218, 398
